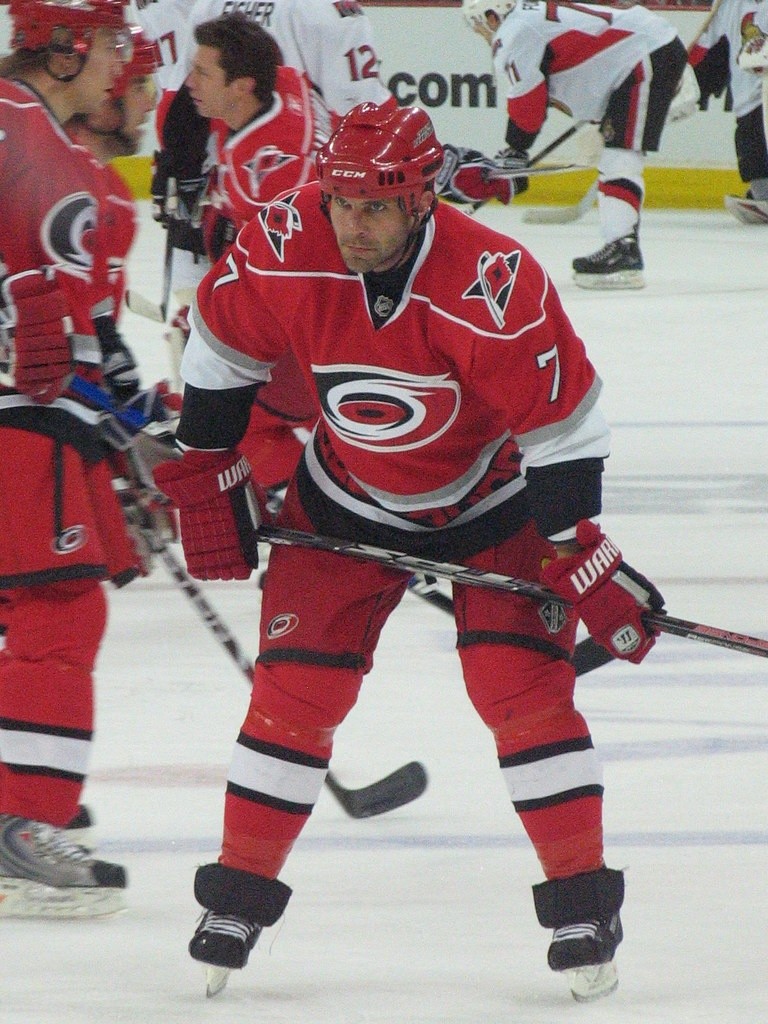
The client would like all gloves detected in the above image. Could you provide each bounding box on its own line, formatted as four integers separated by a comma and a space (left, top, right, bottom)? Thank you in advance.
485, 147, 530, 204
1, 269, 71, 407
537, 521, 668, 665
151, 448, 266, 582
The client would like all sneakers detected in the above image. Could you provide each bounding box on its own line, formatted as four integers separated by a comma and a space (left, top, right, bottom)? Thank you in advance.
572, 232, 644, 289
548, 910, 623, 1003
0, 805, 126, 920
188, 908, 262, 999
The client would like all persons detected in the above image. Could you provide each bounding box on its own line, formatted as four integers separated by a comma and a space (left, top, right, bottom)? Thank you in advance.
1, 0, 768, 923
155, 98, 656, 1001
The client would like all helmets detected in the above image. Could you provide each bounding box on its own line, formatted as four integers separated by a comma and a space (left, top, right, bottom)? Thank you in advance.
7, 0, 132, 62
104, 25, 161, 105
462, 0, 517, 47
315, 101, 446, 217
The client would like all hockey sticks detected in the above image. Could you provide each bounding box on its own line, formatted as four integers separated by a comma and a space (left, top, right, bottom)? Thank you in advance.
254, 521, 768, 658
464, 121, 589, 221
114, 457, 427, 820
